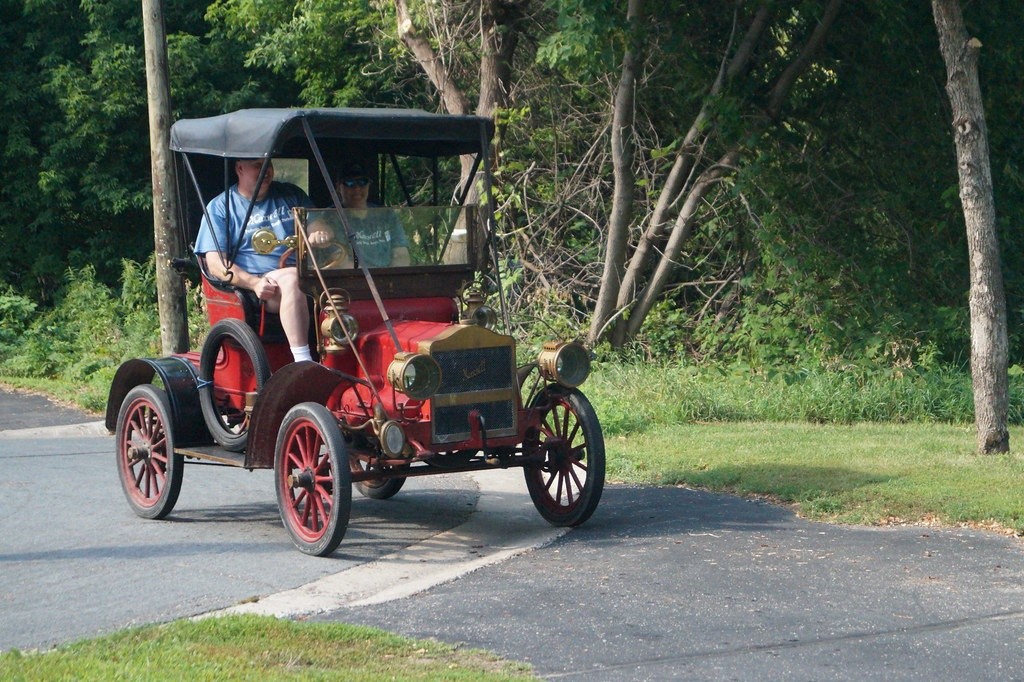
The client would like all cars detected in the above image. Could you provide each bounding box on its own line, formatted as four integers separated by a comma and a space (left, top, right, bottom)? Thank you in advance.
103, 107, 607, 557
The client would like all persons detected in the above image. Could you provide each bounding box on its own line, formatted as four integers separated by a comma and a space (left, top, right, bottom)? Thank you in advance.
320, 166, 411, 267
193, 158, 334, 363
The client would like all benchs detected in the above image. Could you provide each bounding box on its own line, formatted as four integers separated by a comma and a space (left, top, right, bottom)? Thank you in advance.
190, 241, 288, 342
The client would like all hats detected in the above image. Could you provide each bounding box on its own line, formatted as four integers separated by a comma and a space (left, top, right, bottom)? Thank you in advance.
336, 161, 374, 184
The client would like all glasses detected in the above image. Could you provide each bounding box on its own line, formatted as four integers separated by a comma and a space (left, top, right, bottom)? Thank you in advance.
344, 178, 369, 188
